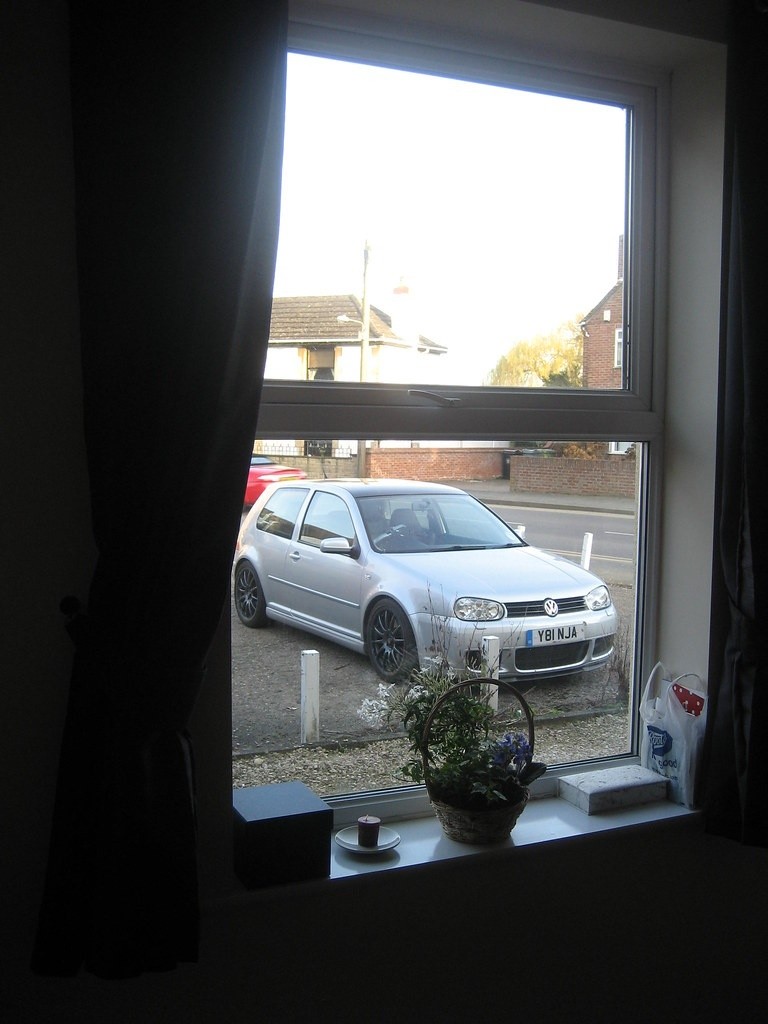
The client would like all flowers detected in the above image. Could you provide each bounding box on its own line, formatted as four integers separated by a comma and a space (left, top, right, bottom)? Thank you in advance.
356, 583, 548, 811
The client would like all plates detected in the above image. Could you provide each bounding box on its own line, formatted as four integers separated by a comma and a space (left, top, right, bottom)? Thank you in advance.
335, 826, 401, 854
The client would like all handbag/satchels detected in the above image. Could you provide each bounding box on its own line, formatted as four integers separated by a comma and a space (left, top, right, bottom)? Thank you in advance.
638, 661, 708, 810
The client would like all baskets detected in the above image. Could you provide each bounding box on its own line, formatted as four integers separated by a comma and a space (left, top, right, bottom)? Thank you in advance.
420, 677, 535, 843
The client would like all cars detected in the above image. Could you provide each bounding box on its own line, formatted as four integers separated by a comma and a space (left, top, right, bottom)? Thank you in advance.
243, 455, 306, 513
234, 477, 617, 683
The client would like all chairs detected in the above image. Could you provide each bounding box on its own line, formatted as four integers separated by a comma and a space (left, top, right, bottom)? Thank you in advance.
390, 508, 425, 536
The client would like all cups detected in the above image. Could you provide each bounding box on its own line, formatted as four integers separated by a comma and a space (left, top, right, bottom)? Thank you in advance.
358, 816, 381, 846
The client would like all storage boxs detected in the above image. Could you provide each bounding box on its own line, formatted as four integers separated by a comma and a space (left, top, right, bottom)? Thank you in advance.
556, 764, 669, 816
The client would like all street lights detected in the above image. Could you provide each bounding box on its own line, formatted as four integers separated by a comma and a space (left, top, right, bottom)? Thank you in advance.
337, 314, 369, 478
